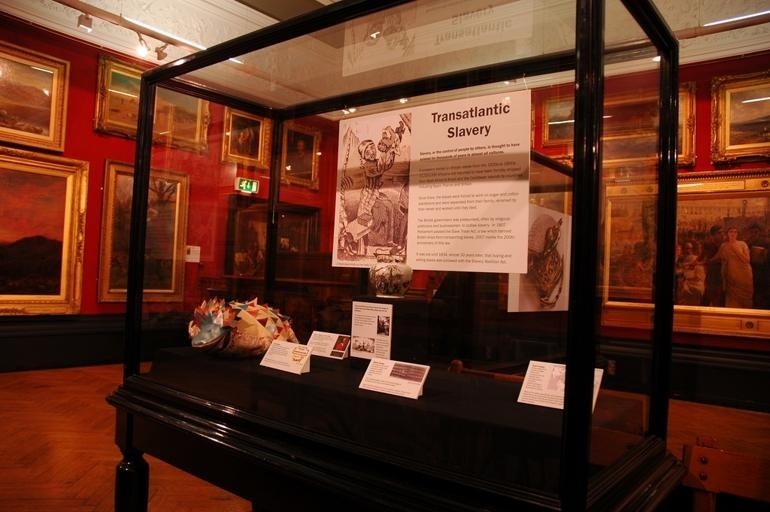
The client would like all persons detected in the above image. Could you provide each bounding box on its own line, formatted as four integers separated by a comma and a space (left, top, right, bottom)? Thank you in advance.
341, 120, 406, 257
335, 337, 347, 350
614, 220, 770, 309
291, 140, 311, 178
235, 240, 263, 276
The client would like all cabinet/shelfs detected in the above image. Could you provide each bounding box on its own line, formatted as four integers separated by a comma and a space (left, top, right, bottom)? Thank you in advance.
106, 1, 689, 511
